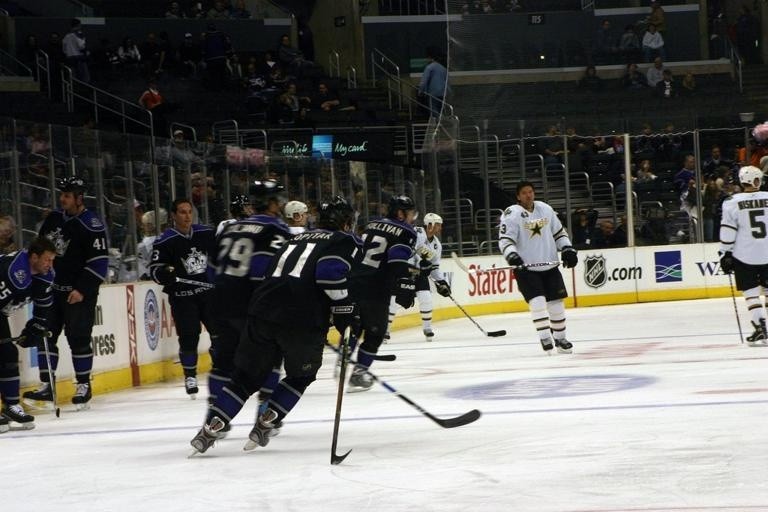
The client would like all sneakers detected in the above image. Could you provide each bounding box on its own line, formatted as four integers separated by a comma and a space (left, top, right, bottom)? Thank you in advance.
23, 383, 59, 401
191, 416, 232, 453
554, 337, 573, 350
4, 404, 34, 423
274, 419, 283, 430
247, 418, 270, 447
184, 373, 199, 394
0, 413, 8, 425
744, 319, 768, 343
423, 325, 435, 337
257, 404, 280, 436
349, 364, 374, 388
383, 328, 393, 341
540, 336, 554, 353
72, 383, 94, 404
332, 360, 345, 378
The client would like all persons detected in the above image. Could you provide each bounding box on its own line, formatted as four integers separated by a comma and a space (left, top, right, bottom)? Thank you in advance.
646, 57, 666, 90
709, 4, 761, 64
167, 2, 187, 20
191, 194, 366, 453
642, 0, 666, 33
190, 3, 212, 21
0, 239, 57, 427
16, 18, 195, 80
417, 54, 447, 123
231, 0, 253, 20
23, 178, 109, 405
597, 20, 618, 63
579, 66, 609, 99
620, 63, 645, 91
456, 0, 526, 19
150, 199, 215, 393
613, 215, 642, 250
214, 193, 255, 236
497, 181, 578, 349
206, 179, 298, 429
572, 208, 599, 250
656, 70, 678, 101
284, 200, 310, 239
680, 132, 768, 218
642, 23, 667, 64
199, 24, 368, 125
678, 70, 701, 99
619, 24, 640, 62
720, 165, 768, 341
385, 211, 449, 338
1, 115, 106, 246
150, 125, 223, 201
517, 121, 679, 197
138, 80, 163, 122
338, 195, 417, 388
209, 0, 231, 19
595, 219, 612, 248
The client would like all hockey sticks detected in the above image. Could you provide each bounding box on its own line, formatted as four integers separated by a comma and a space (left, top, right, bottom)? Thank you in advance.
43, 338, 61, 419
452, 253, 566, 273
349, 339, 396, 361
428, 272, 505, 338
325, 340, 481, 428
331, 328, 351, 466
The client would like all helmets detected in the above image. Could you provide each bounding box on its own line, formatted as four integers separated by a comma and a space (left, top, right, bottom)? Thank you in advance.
284, 200, 308, 221
424, 212, 444, 232
230, 194, 249, 215
752, 124, 768, 140
316, 195, 354, 229
57, 175, 88, 198
249, 175, 289, 198
388, 194, 415, 221
738, 165, 763, 188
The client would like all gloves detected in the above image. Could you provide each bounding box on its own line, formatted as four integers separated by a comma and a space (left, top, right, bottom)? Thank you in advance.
506, 252, 524, 268
721, 252, 735, 277
436, 279, 451, 298
17, 319, 47, 348
395, 281, 418, 310
560, 247, 579, 268
334, 305, 362, 335
157, 265, 177, 286
